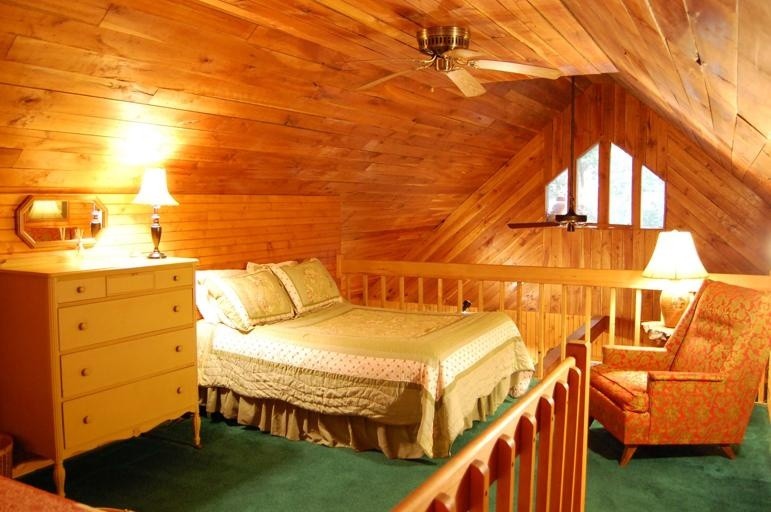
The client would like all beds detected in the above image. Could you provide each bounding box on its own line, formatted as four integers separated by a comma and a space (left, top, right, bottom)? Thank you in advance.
185, 257, 539, 464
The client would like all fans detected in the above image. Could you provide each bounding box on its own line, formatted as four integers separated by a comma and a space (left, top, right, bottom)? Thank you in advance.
338, 23, 564, 99
508, 77, 635, 235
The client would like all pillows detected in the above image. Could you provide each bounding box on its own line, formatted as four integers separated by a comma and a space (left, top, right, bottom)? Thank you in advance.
198, 269, 297, 336
262, 256, 344, 321
244, 259, 300, 274
194, 267, 246, 329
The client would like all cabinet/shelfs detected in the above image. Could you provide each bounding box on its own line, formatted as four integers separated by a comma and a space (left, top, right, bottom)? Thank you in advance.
1, 248, 205, 502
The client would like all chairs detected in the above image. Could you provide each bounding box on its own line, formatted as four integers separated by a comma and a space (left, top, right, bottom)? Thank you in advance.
584, 277, 770, 470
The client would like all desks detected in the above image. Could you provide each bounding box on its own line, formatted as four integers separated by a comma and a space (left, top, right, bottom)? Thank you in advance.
642, 320, 673, 346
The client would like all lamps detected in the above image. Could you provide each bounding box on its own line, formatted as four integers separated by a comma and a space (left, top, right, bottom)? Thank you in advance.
641, 226, 708, 326
132, 162, 178, 262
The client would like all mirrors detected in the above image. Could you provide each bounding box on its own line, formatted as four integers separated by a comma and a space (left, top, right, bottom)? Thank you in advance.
14, 194, 109, 250
